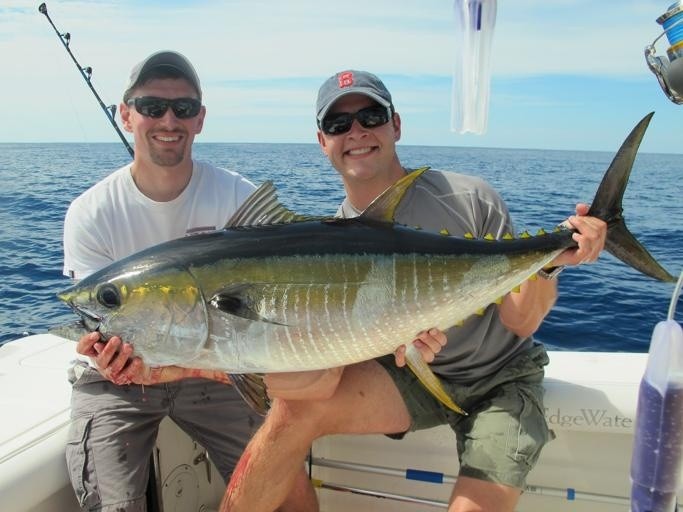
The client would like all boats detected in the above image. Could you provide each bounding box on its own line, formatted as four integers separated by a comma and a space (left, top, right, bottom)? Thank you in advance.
1, 322, 683, 511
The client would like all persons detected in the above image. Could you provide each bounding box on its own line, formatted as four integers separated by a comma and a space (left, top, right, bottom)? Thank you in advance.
74, 69, 611, 511
59, 48, 322, 511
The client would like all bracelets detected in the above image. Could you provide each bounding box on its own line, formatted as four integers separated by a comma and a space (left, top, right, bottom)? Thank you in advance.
538, 266, 566, 281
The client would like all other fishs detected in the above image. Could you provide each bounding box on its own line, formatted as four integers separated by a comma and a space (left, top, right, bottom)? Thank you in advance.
56, 111, 678, 417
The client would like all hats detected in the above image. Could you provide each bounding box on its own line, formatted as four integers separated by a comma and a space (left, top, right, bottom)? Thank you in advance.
123, 50, 202, 100
315, 70, 394, 128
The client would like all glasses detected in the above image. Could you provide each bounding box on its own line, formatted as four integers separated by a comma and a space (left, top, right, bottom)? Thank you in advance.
321, 105, 392, 134
127, 96, 200, 118
